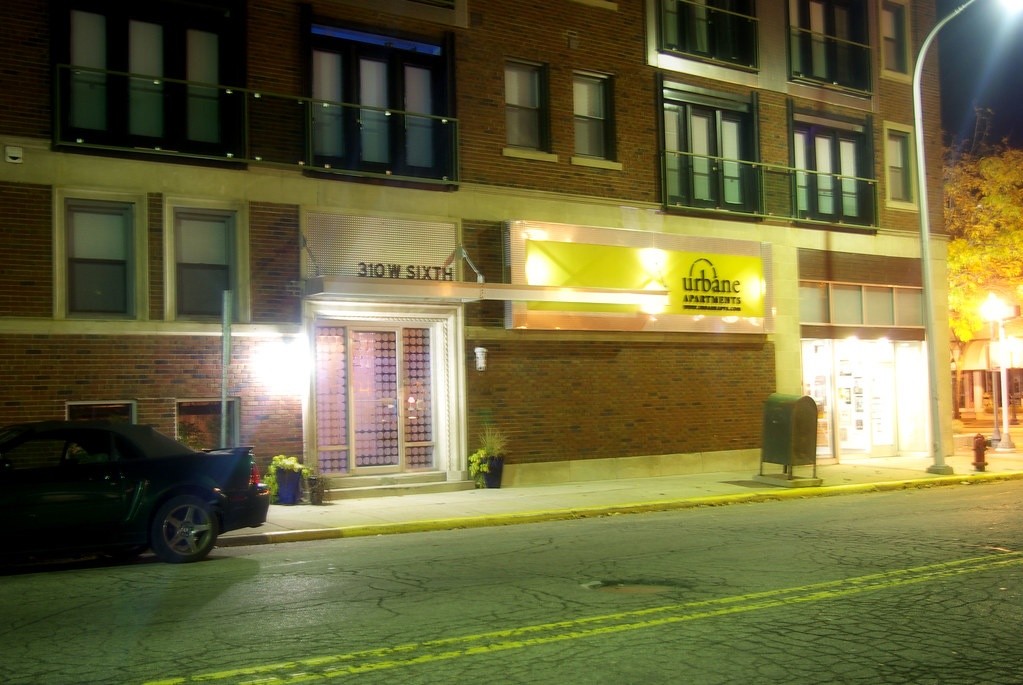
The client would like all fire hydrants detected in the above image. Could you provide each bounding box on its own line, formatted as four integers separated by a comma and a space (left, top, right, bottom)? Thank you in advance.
972, 432, 990, 473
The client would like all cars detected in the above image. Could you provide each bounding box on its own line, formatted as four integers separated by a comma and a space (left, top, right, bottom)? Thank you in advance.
0, 419, 273, 562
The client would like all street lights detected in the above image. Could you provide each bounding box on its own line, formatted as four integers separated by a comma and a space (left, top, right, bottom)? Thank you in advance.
977, 292, 1017, 451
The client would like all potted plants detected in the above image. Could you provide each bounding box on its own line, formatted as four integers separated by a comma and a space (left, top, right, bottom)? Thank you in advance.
264, 456, 331, 507
466, 423, 512, 490
